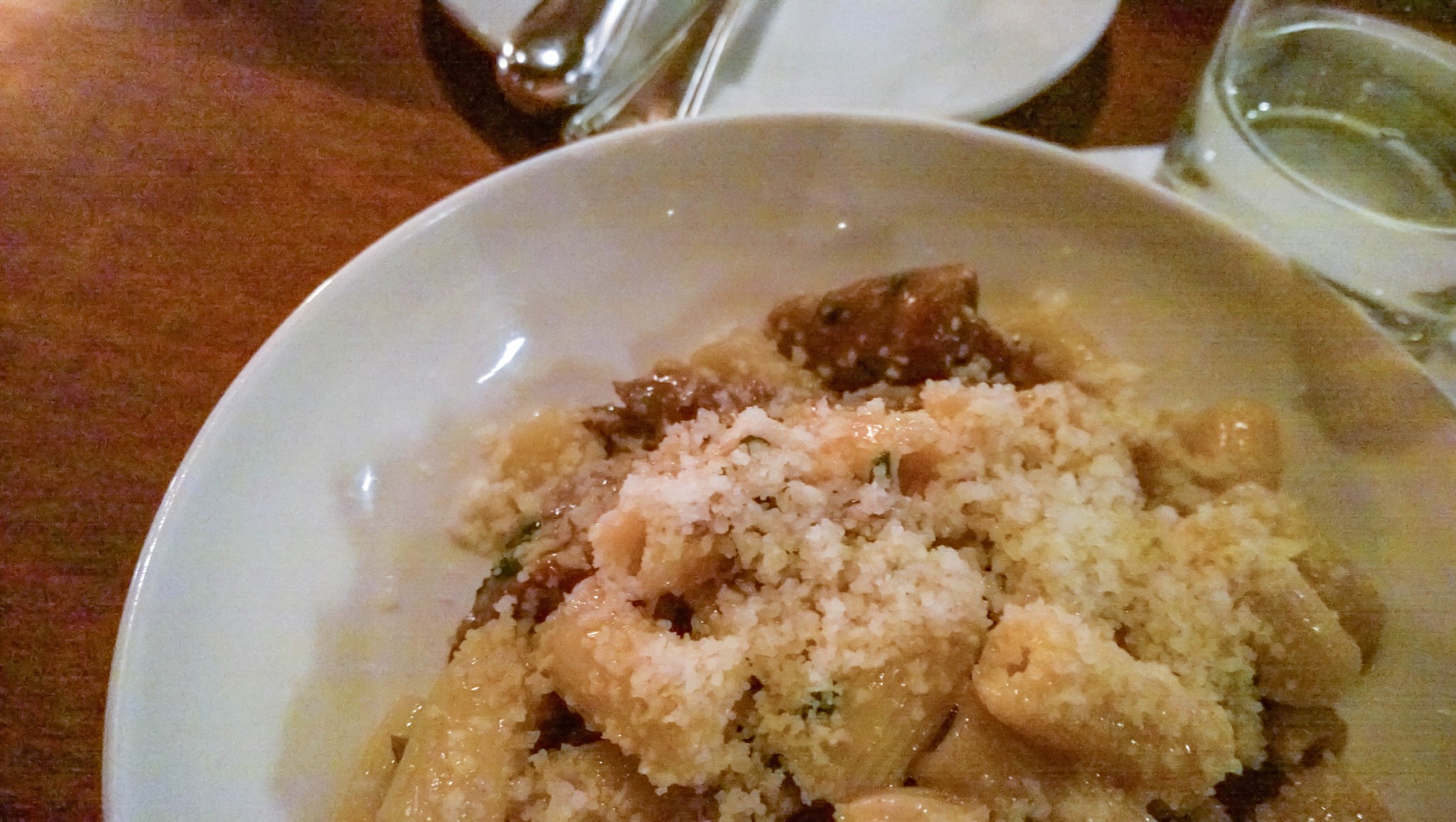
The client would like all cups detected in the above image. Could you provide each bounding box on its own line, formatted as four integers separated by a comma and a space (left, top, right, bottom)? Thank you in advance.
1151, 0, 1455, 362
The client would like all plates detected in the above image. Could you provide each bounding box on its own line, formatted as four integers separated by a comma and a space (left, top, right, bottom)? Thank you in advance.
436, 1, 1118, 138
101, 108, 1454, 822
